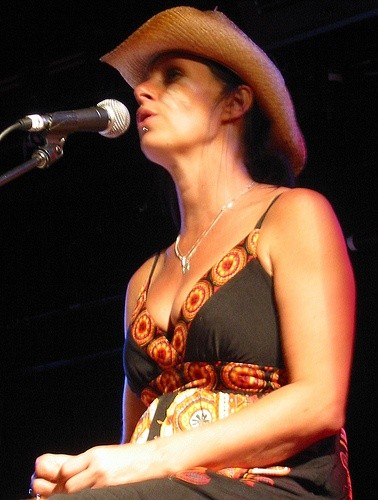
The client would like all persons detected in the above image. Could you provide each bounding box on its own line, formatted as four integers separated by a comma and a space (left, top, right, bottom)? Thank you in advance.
26, 6, 354, 499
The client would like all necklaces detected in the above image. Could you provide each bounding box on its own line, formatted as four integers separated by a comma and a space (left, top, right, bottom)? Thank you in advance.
174, 180, 256, 274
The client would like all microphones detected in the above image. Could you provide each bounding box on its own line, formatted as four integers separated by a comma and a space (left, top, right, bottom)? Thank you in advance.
18, 97, 131, 140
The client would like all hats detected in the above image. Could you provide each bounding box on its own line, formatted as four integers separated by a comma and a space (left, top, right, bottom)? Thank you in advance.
103, 0, 306, 178
